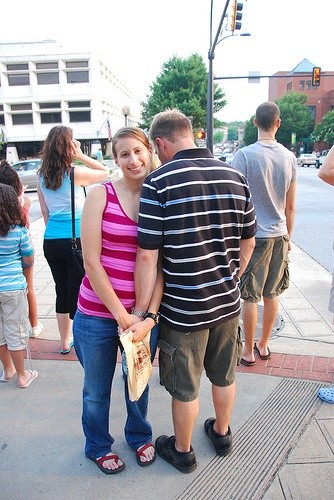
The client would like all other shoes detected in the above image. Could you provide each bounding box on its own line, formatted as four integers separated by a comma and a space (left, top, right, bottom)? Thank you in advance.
30, 323, 44, 338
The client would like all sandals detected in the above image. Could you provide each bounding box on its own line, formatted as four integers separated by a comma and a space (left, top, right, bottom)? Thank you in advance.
318, 388, 334, 404
89, 451, 126, 475
135, 442, 157, 467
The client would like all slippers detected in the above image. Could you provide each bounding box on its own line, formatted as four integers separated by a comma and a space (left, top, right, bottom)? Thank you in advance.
239, 350, 256, 365
18, 369, 38, 388
61, 337, 74, 354
0, 364, 17, 382
254, 339, 271, 360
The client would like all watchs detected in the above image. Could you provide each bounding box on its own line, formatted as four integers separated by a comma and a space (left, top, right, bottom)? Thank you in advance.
144, 312, 160, 325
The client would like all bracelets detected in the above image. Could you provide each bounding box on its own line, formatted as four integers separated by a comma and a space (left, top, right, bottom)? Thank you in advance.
131, 310, 147, 317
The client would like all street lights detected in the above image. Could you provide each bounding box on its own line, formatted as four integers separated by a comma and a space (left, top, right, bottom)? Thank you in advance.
122, 106, 131, 127
205, 32, 252, 156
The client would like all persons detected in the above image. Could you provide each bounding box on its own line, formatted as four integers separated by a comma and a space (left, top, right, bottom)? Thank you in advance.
72, 127, 165, 475
232, 101, 296, 367
117, 111, 258, 475
0, 158, 44, 337
318, 144, 334, 405
0, 183, 39, 388
37, 125, 110, 354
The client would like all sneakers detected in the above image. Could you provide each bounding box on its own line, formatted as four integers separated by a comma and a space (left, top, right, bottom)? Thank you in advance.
205, 417, 232, 455
155, 435, 197, 473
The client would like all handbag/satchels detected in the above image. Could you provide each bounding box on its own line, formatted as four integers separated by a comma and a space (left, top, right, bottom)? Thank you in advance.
71, 250, 85, 275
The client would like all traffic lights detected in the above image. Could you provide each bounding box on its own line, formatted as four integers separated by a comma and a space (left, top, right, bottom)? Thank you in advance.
231, 0, 243, 32
195, 130, 206, 141
312, 66, 321, 86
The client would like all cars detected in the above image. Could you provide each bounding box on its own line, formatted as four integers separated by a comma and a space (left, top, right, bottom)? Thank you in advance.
213, 142, 241, 162
315, 152, 328, 169
9, 159, 42, 193
298, 154, 318, 167
